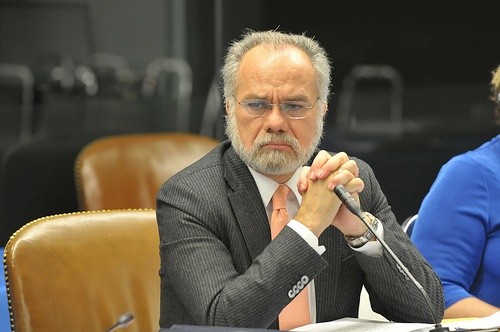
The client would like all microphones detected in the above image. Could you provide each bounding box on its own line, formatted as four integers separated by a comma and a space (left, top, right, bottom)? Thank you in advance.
334, 184, 450, 332
106, 312, 135, 332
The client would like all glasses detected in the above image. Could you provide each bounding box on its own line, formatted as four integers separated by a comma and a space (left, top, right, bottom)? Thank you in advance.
232, 95, 320, 120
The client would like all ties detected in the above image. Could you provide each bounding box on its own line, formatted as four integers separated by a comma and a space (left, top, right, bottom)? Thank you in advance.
271, 184, 310, 332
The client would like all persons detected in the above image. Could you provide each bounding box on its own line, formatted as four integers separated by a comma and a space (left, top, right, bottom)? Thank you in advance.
408, 67, 500, 319
154, 25, 445, 331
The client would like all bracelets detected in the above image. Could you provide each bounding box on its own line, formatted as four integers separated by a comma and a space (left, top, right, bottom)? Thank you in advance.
343, 211, 378, 248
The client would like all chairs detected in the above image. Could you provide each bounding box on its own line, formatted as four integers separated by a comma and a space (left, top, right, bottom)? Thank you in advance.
2, 205, 161, 332
75, 133, 221, 210
